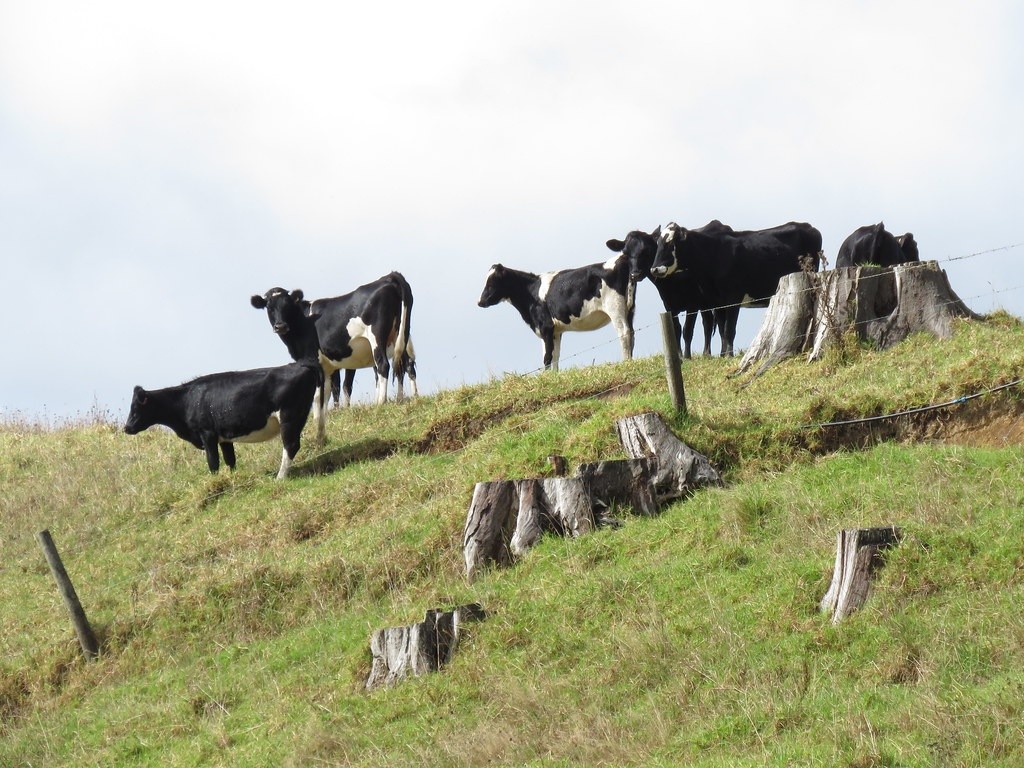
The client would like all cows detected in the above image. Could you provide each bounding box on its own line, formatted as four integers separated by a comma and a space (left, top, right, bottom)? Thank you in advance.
477, 262, 636, 370
124, 356, 324, 480
606, 221, 822, 358
835, 222, 919, 266
249, 269, 417, 408
